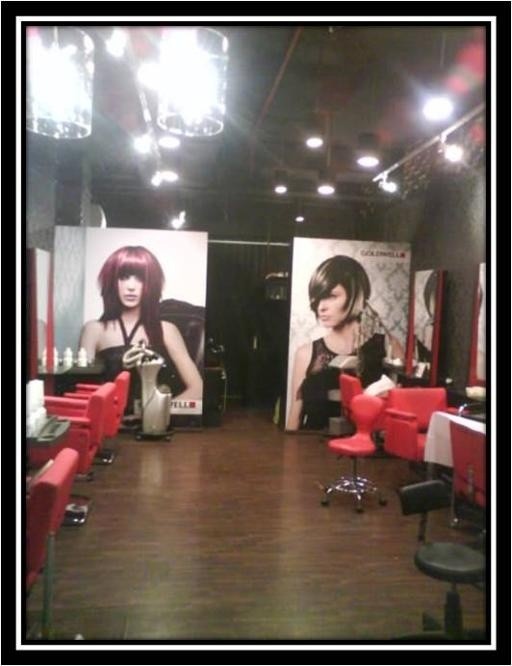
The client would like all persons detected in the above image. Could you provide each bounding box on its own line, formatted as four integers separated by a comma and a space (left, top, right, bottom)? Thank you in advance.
413, 270, 438, 380
286, 255, 406, 432
79, 246, 203, 401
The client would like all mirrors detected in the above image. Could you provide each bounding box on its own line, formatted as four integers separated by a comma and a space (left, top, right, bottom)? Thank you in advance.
465, 261, 487, 386
404, 270, 446, 387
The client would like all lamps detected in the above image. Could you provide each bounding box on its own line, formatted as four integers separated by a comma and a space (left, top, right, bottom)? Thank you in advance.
153, 23, 229, 138
24, 24, 97, 142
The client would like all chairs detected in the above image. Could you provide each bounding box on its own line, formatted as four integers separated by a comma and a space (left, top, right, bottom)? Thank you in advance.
21, 371, 131, 642
319, 372, 486, 641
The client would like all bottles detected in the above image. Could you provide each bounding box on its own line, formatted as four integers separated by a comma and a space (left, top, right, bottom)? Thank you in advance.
53, 347, 88, 371
